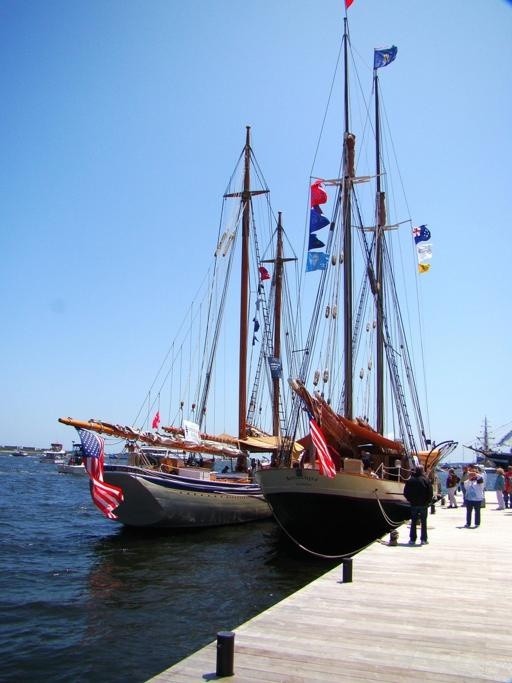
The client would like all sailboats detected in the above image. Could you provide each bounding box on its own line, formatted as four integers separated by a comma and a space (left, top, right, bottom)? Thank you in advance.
252, 0, 455, 564
462, 414, 512, 470
55, 118, 305, 544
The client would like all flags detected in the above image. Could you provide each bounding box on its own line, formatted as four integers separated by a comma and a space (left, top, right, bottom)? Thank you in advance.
304, 180, 330, 272
374, 47, 398, 69
308, 412, 336, 478
259, 267, 270, 280
76, 426, 124, 520
153, 411, 160, 428
413, 225, 432, 273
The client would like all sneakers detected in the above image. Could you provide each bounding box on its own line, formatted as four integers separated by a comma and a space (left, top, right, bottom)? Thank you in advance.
408, 540, 429, 544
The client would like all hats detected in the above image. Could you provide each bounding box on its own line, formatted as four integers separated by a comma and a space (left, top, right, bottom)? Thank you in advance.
415, 465, 424, 474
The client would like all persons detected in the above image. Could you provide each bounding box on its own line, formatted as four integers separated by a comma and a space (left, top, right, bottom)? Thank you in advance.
446, 464, 488, 527
360, 450, 370, 470
404, 465, 433, 545
494, 466, 512, 510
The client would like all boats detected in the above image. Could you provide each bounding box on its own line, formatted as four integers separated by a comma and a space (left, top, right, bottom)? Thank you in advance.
10, 443, 128, 476
